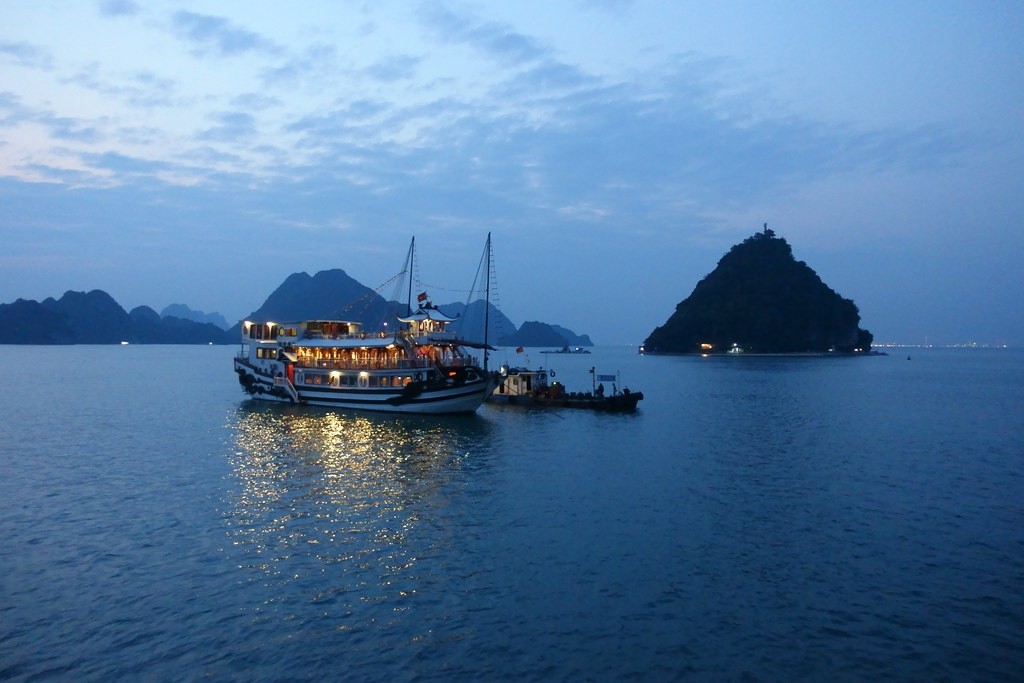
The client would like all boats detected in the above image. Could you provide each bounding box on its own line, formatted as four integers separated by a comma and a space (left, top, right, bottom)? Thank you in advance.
487, 366, 643, 408
234, 230, 510, 413
540, 344, 590, 354
